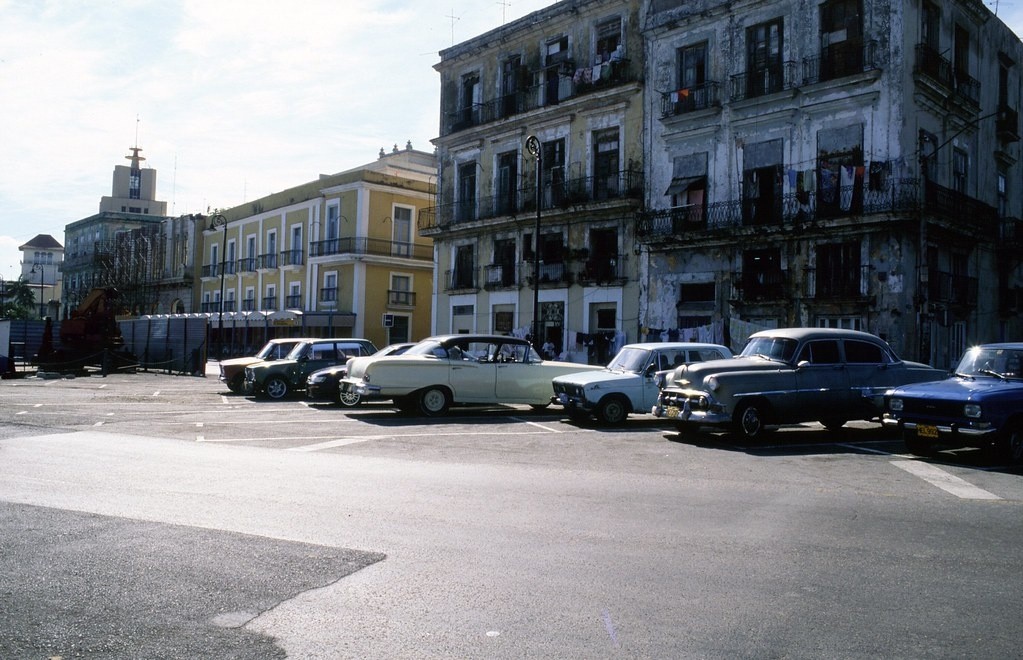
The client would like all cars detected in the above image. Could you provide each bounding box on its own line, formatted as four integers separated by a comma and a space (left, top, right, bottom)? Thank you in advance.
883, 342, 1023, 462
551, 341, 735, 432
304, 341, 479, 410
339, 333, 608, 420
652, 327, 950, 447
218, 336, 322, 393
241, 337, 382, 403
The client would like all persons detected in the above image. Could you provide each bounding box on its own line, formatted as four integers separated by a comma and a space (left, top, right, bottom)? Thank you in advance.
542, 337, 556, 360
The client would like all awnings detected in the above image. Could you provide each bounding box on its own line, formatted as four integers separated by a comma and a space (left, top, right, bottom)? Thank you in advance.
140, 309, 303, 327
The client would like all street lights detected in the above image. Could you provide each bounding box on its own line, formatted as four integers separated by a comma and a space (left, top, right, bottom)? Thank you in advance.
29, 262, 44, 321
207, 212, 228, 365
